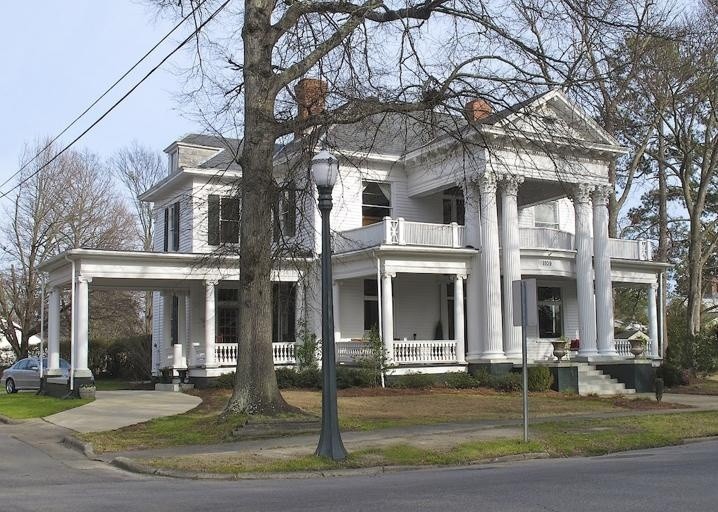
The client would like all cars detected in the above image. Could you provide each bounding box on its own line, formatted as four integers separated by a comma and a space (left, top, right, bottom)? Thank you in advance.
2, 357, 72, 394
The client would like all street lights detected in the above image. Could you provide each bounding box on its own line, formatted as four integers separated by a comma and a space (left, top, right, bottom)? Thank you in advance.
309, 148, 351, 463
